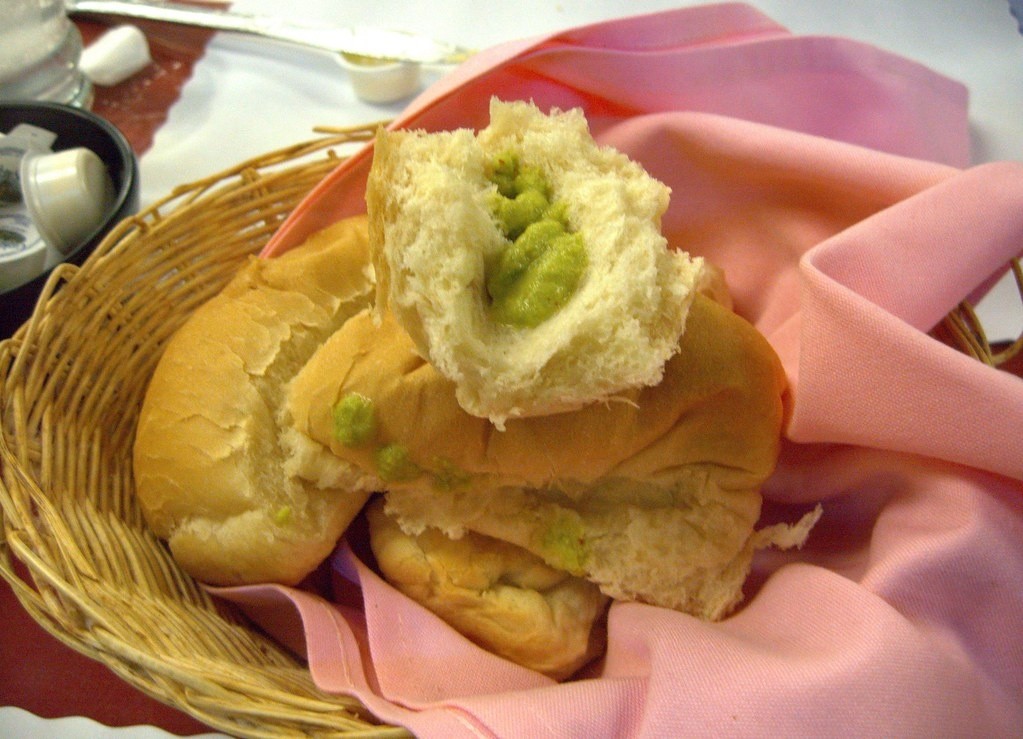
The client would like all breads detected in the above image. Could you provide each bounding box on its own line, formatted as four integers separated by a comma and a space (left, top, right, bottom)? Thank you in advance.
132, 97, 795, 678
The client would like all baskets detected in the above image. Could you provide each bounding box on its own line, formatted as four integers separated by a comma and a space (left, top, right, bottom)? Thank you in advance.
0, 121, 1023, 739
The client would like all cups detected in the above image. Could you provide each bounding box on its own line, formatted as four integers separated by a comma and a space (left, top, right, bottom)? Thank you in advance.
340, 30, 424, 102
1, 0, 90, 111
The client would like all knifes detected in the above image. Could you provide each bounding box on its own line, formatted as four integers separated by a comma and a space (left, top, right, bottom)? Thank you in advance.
66, 0, 448, 66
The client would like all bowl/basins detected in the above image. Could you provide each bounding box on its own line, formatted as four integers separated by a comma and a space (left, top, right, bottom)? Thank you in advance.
0, 97, 146, 337
